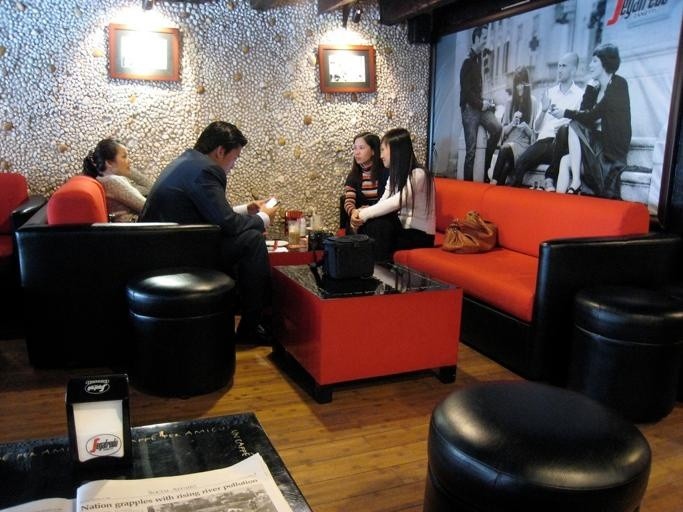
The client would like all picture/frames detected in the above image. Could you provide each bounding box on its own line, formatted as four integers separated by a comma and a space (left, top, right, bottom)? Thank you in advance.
108, 24, 182, 82
318, 43, 377, 93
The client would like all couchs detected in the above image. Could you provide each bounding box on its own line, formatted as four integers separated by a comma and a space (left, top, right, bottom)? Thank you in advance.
15, 172, 223, 374
0, 172, 48, 340
337, 177, 683, 382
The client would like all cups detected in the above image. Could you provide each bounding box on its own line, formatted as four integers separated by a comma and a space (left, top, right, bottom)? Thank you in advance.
288, 220, 300, 246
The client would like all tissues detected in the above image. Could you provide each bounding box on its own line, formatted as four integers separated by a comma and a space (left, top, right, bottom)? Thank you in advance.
64, 372, 133, 473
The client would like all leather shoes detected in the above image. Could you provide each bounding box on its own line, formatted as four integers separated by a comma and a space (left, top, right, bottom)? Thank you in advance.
234, 318, 274, 347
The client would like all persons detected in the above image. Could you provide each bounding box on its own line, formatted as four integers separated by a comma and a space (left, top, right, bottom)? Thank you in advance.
344, 132, 380, 236
490, 64, 538, 186
506, 53, 584, 190
82, 138, 155, 222
548, 43, 632, 200
138, 122, 279, 347
460, 24, 502, 184
351, 128, 436, 292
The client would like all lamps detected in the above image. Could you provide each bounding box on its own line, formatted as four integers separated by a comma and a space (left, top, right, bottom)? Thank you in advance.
142, 0, 153, 12
352, 6, 363, 23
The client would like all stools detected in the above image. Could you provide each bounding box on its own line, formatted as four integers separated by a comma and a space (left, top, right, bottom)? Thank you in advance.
126, 270, 236, 400
571, 288, 683, 426
421, 381, 651, 512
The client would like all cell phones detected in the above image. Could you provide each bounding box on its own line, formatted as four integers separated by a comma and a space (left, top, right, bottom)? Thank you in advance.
266, 199, 279, 208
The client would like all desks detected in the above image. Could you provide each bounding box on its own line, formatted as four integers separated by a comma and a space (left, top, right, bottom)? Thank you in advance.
0, 413, 313, 512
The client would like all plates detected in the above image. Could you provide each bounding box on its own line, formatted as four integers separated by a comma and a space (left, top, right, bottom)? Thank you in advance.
265, 240, 288, 246
268, 247, 288, 253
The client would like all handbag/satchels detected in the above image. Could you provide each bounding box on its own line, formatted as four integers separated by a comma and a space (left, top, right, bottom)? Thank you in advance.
308, 234, 375, 287
442, 210, 497, 253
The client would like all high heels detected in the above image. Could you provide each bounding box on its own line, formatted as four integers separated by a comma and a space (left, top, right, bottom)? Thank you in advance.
565, 185, 582, 195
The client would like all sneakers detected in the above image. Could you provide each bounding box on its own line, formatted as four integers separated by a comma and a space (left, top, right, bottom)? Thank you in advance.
545, 179, 555, 192
